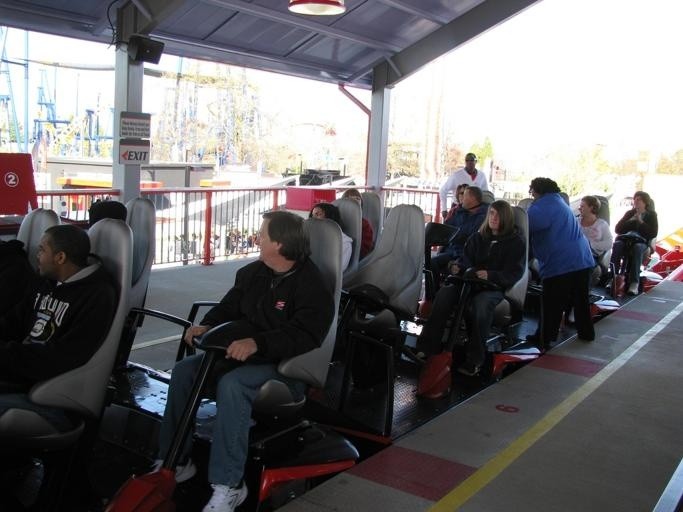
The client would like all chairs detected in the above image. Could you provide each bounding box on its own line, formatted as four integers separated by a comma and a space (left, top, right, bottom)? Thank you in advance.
18, 209, 65, 271
2, 218, 133, 443
121, 195, 166, 307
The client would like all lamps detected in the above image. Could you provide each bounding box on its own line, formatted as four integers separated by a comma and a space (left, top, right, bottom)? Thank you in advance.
289, 0, 344, 16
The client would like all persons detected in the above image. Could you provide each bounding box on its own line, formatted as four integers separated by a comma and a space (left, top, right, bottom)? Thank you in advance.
149, 210, 337, 512
0, 220, 111, 430
525, 176, 597, 346
417, 186, 491, 319
436, 182, 469, 254
440, 152, 488, 219
399, 199, 528, 377
603, 190, 659, 295
0, 239, 37, 343
557, 194, 615, 333
84, 149, 373, 277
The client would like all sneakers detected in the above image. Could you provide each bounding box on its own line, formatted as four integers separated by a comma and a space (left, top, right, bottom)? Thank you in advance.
202, 481, 248, 512
153, 460, 196, 482
627, 282, 639, 295
